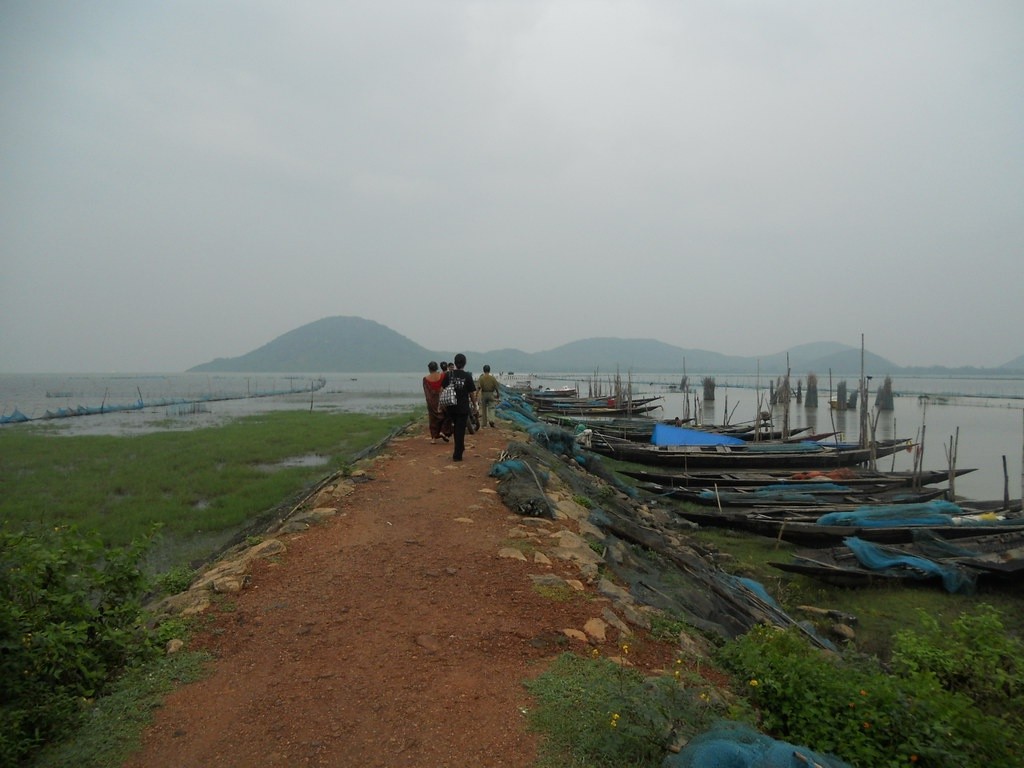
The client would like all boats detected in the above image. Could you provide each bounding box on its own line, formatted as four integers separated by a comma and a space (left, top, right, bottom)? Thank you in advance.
767, 530, 1024, 590
635, 485, 953, 508
828, 395, 850, 410
673, 499, 1024, 530
727, 520, 1024, 548
517, 390, 920, 468
615, 468, 978, 489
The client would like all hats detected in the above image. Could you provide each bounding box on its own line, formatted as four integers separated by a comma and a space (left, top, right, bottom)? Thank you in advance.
428, 361, 438, 370
440, 362, 447, 368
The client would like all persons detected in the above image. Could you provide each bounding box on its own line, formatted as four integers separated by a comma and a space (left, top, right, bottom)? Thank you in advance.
422, 353, 478, 462
475, 364, 501, 427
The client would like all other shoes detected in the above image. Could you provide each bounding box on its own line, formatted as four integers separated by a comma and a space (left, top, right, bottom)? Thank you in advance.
430, 440, 436, 444
489, 421, 496, 428
439, 432, 449, 442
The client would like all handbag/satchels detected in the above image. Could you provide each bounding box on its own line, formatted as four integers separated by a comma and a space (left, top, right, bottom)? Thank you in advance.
438, 384, 457, 413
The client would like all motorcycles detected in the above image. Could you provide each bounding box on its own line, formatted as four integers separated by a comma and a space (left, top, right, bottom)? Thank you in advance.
467, 397, 479, 434
